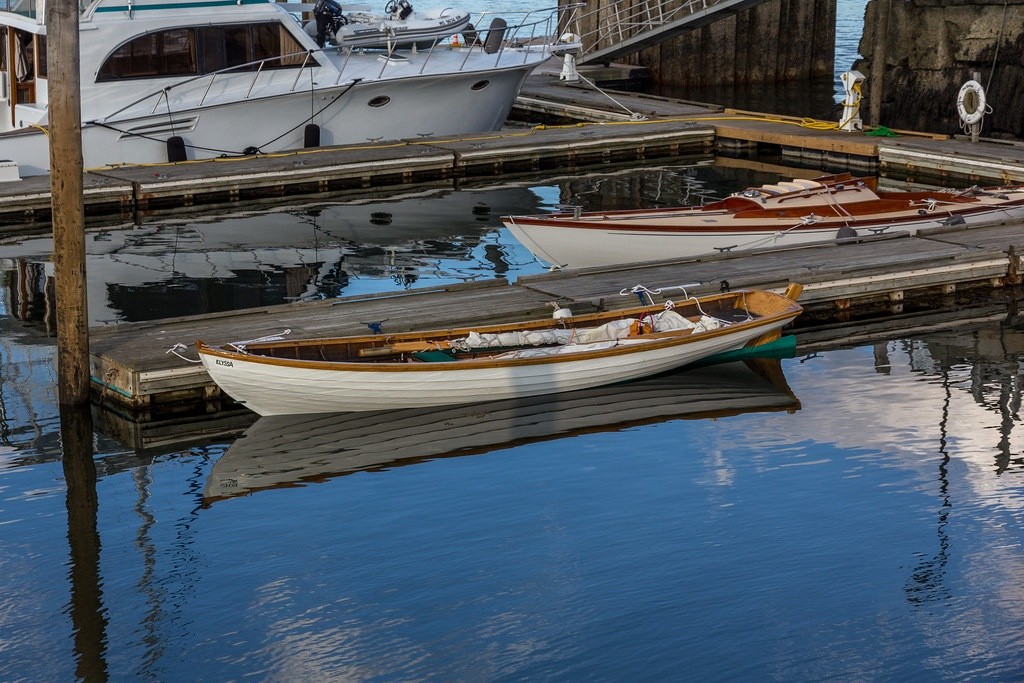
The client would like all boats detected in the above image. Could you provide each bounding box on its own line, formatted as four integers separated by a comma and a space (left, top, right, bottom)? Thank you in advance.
196, 283, 805, 418
0, 151, 549, 342
500, 172, 1023, 272
200, 328, 802, 511
0, 0, 588, 182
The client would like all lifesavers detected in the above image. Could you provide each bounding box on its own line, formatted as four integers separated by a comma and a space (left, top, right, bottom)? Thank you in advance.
957, 80, 986, 124
